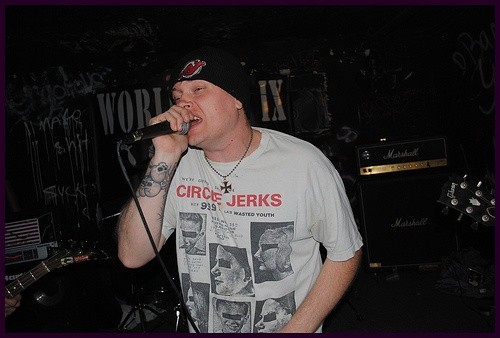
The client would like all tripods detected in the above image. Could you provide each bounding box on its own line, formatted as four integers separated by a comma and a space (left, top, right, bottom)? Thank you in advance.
119, 268, 170, 333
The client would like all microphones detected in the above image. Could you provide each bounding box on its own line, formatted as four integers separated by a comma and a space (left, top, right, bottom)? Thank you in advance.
123, 119, 191, 145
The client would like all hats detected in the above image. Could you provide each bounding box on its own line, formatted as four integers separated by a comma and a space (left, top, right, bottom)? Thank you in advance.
170, 51, 255, 118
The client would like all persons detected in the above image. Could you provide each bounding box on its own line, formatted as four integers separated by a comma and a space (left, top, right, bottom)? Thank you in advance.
4, 292, 21, 315
116, 52, 363, 335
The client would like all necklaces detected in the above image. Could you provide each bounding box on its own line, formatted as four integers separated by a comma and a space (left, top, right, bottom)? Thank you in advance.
203, 127, 254, 193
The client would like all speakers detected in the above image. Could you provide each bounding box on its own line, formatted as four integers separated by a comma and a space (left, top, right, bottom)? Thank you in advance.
355, 137, 460, 272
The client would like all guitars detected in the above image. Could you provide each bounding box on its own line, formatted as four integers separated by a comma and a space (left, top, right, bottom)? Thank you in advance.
5, 250, 111, 310
436, 169, 496, 233
